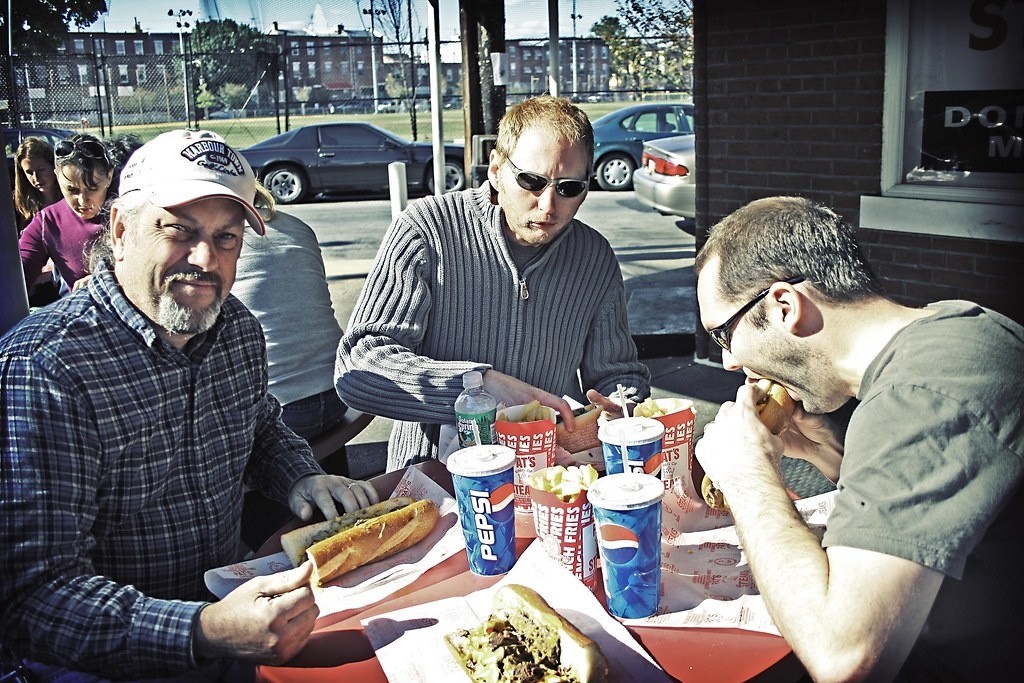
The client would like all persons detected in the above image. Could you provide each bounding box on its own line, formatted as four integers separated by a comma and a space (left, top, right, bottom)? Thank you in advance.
694, 196, 1024, 683
234, 182, 345, 446
13, 134, 142, 302
334, 98, 654, 473
0, 129, 381, 683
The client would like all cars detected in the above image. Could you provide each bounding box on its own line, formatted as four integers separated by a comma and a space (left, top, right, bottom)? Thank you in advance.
631, 133, 696, 231
3, 127, 75, 192
572, 93, 615, 103
589, 103, 695, 192
377, 98, 463, 113
238, 122, 465, 205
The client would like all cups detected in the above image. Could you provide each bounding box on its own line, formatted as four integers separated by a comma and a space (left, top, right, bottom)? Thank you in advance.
633, 398, 695, 494
446, 445, 516, 577
528, 468, 599, 593
586, 471, 666, 618
597, 417, 665, 484
493, 405, 557, 516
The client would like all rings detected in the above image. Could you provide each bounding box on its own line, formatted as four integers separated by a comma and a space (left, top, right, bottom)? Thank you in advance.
347, 482, 358, 490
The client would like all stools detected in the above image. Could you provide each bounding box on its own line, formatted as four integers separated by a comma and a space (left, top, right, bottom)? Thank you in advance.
312, 409, 378, 479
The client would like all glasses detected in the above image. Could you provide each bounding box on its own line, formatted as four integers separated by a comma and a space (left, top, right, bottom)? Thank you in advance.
709, 276, 810, 351
503, 156, 595, 199
55, 140, 107, 159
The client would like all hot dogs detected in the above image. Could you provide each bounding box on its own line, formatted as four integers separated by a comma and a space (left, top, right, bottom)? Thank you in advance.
701, 379, 795, 511
444, 584, 606, 683
279, 495, 438, 585
556, 404, 604, 454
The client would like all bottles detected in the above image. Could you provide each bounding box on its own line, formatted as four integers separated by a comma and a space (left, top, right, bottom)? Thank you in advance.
455, 372, 496, 448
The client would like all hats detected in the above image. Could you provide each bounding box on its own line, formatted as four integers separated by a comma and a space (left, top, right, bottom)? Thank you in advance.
119, 129, 267, 237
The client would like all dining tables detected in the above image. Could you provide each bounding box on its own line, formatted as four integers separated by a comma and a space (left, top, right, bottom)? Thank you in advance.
253, 483, 801, 683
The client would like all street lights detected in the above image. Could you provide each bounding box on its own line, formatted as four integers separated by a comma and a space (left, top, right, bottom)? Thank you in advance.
571, 13, 582, 97
168, 8, 193, 130
362, 7, 388, 114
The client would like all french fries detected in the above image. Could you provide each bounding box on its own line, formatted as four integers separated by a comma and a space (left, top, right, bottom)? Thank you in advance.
532, 464, 594, 502
637, 397, 684, 418
499, 399, 552, 423
